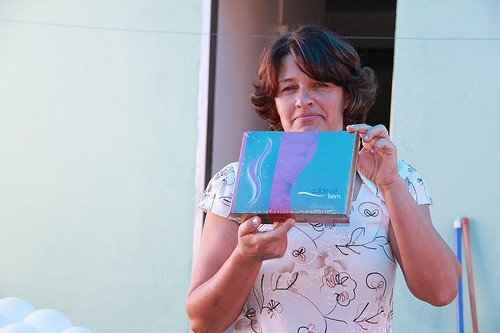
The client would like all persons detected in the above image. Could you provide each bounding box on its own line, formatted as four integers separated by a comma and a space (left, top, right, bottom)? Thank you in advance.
186, 23, 462, 333
